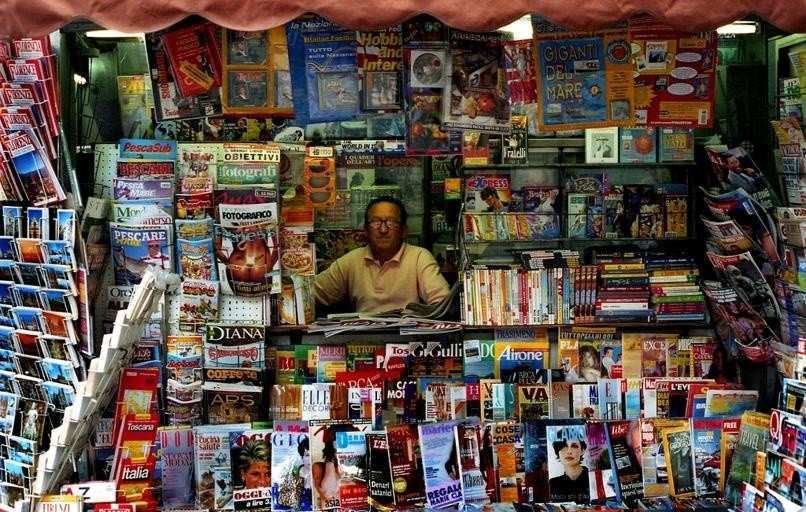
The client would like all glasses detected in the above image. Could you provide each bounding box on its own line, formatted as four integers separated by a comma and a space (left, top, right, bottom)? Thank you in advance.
368, 219, 402, 229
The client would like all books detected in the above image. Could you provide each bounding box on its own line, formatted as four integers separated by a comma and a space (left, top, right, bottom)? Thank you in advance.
308, 280, 465, 510
434, 23, 806, 511
0, 22, 315, 511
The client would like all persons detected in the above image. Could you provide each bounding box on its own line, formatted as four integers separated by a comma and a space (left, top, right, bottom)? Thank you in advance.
315, 195, 452, 313
